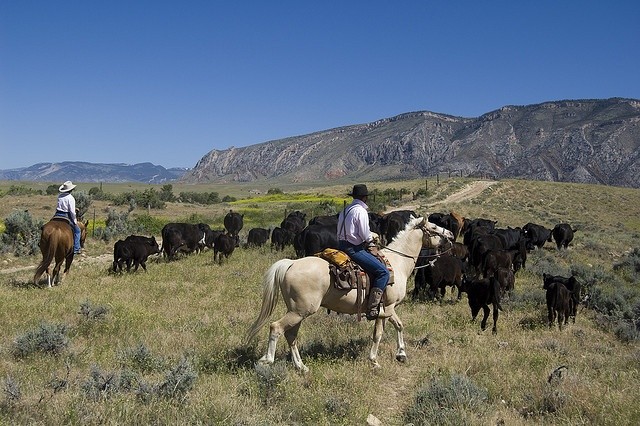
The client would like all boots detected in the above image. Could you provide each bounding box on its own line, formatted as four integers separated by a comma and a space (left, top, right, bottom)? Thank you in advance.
366, 288, 392, 320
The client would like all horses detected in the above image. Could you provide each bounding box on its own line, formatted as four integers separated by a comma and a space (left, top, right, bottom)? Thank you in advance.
33, 216, 90, 289
242, 211, 456, 376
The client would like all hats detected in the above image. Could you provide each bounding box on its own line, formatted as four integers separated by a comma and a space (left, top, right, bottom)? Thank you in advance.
58, 180, 77, 192
347, 184, 374, 197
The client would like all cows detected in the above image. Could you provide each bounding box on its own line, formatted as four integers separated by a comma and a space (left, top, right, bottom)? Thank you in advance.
159, 211, 244, 274
245, 227, 271, 249
111, 231, 161, 275
552, 223, 578, 251
542, 272, 581, 332
270, 210, 553, 337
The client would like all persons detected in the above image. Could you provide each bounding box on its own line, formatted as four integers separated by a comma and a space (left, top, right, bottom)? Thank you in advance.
336, 184, 392, 320
54, 180, 82, 254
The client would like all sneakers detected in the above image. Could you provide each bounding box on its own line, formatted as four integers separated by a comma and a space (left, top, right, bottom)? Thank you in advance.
74, 250, 83, 254
